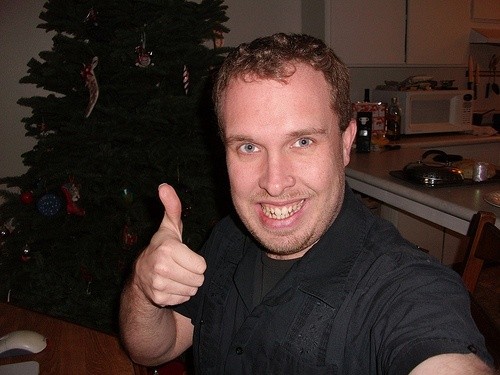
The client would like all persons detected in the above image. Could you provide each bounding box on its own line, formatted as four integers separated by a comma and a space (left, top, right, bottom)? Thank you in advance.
118, 31, 497, 375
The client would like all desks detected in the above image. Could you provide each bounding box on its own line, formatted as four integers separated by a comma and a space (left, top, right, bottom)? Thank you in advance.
344, 142, 499, 267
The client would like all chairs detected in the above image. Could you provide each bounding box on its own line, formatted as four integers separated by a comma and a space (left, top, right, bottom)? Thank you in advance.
458, 211, 500, 335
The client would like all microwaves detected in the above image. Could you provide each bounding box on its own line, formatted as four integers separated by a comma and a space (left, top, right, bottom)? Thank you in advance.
401, 90, 474, 134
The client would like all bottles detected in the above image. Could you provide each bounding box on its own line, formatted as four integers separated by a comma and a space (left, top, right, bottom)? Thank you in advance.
385, 97, 403, 142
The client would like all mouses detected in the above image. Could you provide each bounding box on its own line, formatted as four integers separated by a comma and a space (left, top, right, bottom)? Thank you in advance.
0, 330, 48, 358
